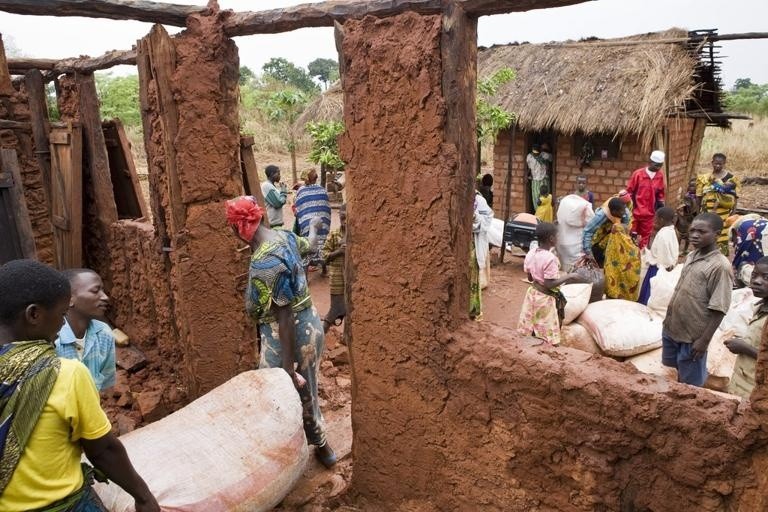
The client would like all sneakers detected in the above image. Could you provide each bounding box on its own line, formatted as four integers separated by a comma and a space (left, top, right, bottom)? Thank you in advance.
315, 446, 336, 467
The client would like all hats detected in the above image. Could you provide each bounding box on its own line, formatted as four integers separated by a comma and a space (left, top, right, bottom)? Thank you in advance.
650, 151, 665, 163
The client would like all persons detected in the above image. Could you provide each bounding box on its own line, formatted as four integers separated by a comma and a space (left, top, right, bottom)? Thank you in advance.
469, 191, 495, 321
0, 259, 159, 512
226, 196, 336, 468
517, 143, 768, 401
57, 268, 115, 393
262, 167, 346, 335
